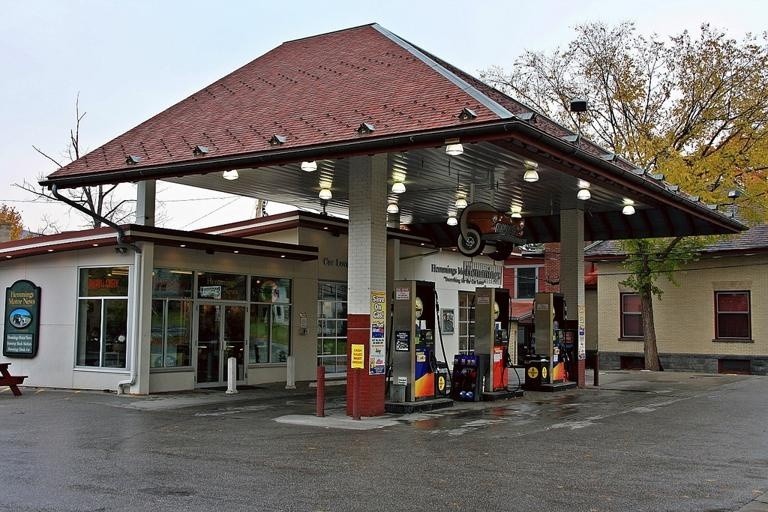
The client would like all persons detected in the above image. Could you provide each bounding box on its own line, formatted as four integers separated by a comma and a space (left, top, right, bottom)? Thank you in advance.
443, 312, 454, 332
272, 289, 280, 302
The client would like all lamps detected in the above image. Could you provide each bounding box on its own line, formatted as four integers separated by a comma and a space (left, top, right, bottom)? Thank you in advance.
577, 189, 591, 200
300, 159, 317, 172
387, 204, 399, 214
318, 190, 332, 200
446, 144, 463, 155
455, 200, 467, 208
623, 206, 635, 215
523, 170, 539, 182
511, 213, 521, 218
222, 170, 239, 181
447, 217, 458, 226
392, 183, 405, 193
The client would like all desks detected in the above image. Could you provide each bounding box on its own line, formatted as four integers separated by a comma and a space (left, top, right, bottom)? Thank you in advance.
0, 362, 24, 395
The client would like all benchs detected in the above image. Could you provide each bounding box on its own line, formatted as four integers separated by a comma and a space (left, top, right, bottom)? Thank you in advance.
0, 375, 28, 386
